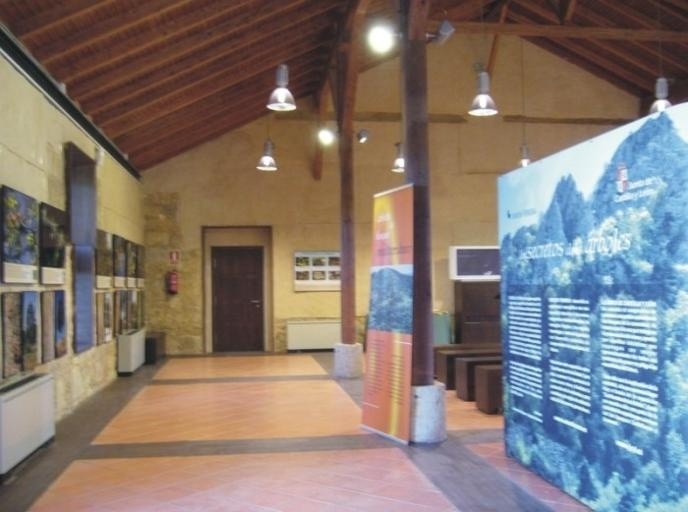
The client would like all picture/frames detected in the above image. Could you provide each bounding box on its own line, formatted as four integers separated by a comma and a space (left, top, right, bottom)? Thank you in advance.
295, 251, 343, 291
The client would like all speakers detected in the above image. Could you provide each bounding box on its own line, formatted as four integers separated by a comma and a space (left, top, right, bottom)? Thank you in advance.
433, 19, 456, 46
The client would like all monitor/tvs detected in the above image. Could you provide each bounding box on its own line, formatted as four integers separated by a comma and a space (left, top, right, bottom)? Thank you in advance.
448, 245, 501, 281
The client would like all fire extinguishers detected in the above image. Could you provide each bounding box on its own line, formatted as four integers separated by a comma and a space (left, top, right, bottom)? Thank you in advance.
167, 268, 178, 294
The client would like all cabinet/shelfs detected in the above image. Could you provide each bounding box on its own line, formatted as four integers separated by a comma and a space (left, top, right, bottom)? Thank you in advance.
118, 329, 146, 375
454, 279, 501, 345
0, 372, 56, 477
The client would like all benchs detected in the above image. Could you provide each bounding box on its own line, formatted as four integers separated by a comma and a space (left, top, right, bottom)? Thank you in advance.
434, 344, 504, 417
287, 319, 345, 350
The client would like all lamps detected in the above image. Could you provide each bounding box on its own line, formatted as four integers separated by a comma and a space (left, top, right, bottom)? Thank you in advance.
466, 0, 499, 117
391, 143, 405, 173
516, 37, 531, 167
648, 0, 672, 115
358, 129, 368, 144
422, 20, 455, 47
266, 63, 296, 112
255, 114, 278, 172
318, 120, 339, 146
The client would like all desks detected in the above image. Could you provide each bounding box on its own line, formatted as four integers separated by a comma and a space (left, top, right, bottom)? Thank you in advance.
146, 331, 167, 363
